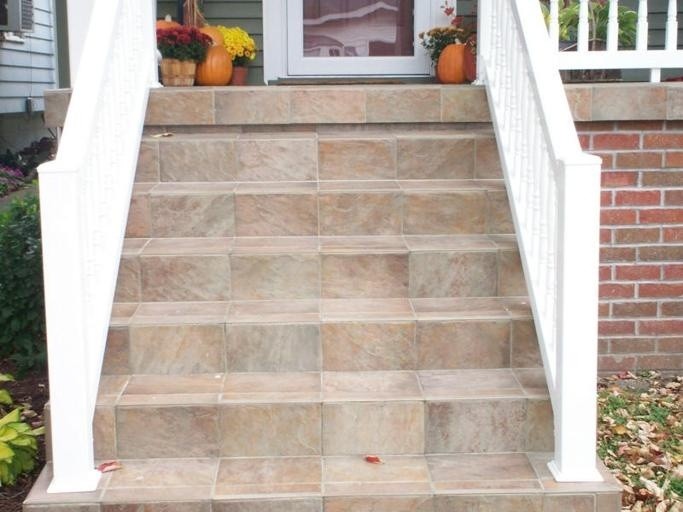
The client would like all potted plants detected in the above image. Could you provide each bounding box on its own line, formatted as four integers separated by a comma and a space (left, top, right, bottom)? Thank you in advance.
539, 1, 638, 83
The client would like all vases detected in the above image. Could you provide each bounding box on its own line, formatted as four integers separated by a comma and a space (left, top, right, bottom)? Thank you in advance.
433, 65, 441, 83
157, 56, 198, 88
229, 64, 250, 86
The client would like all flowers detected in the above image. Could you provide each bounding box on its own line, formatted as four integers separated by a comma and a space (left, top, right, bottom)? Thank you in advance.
214, 22, 257, 69
439, 0, 476, 63
417, 27, 466, 66
155, 18, 213, 66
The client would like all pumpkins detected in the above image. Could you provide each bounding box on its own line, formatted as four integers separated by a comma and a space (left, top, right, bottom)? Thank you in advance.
438, 38, 465, 83
200, 21, 226, 46
157, 14, 181, 30
195, 40, 233, 87
